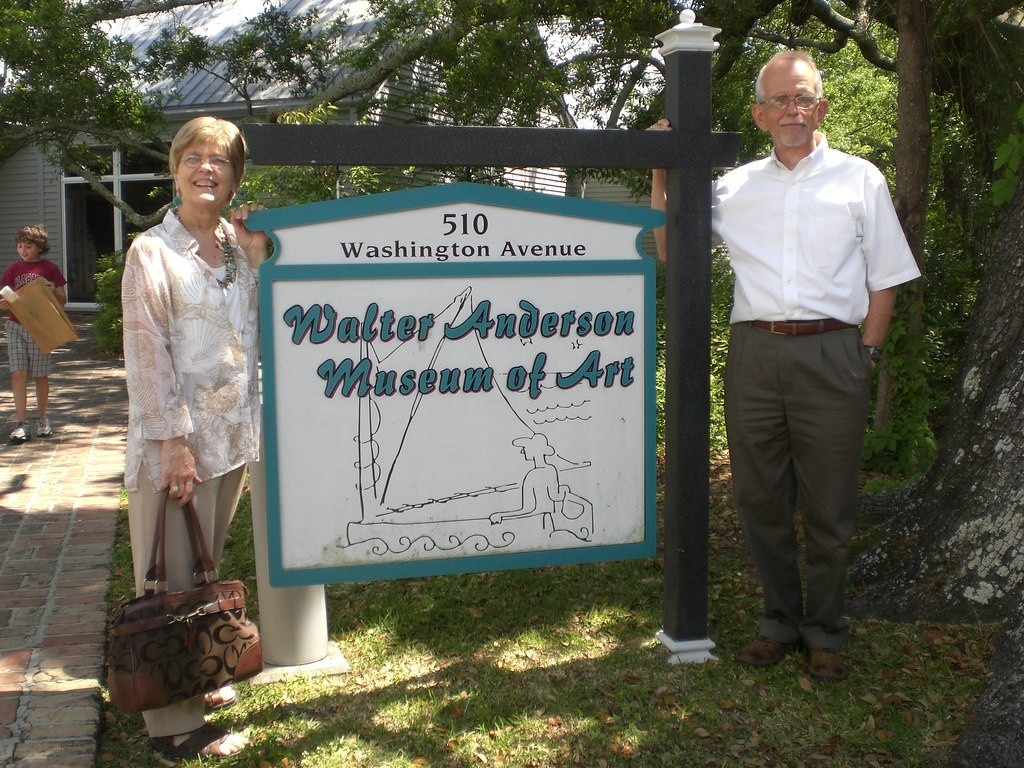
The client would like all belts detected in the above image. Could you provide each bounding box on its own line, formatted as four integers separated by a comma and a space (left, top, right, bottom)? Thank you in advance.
751, 319, 856, 337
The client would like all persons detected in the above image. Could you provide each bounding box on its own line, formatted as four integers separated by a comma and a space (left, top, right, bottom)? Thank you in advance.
117, 115, 270, 761
0, 224, 68, 443
641, 49, 924, 680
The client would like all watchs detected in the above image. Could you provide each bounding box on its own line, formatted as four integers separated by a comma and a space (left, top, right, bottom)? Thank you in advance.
863, 345, 883, 363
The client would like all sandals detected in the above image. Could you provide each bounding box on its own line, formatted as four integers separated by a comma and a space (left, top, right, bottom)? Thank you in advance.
149, 722, 250, 760
203, 685, 237, 714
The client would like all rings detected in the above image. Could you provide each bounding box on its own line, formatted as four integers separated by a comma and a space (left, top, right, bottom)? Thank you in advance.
169, 486, 179, 493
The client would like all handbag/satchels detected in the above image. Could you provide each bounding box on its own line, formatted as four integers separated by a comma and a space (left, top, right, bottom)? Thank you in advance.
5, 278, 79, 354
105, 487, 263, 712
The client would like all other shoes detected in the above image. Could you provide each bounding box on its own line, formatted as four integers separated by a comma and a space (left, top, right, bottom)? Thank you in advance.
36, 416, 53, 436
739, 636, 785, 665
809, 648, 845, 679
9, 420, 31, 442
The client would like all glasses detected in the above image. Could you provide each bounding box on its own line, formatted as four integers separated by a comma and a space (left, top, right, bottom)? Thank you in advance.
759, 96, 822, 110
180, 154, 231, 171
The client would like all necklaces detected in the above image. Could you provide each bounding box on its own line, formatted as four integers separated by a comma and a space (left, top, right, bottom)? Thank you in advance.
175, 202, 238, 289
